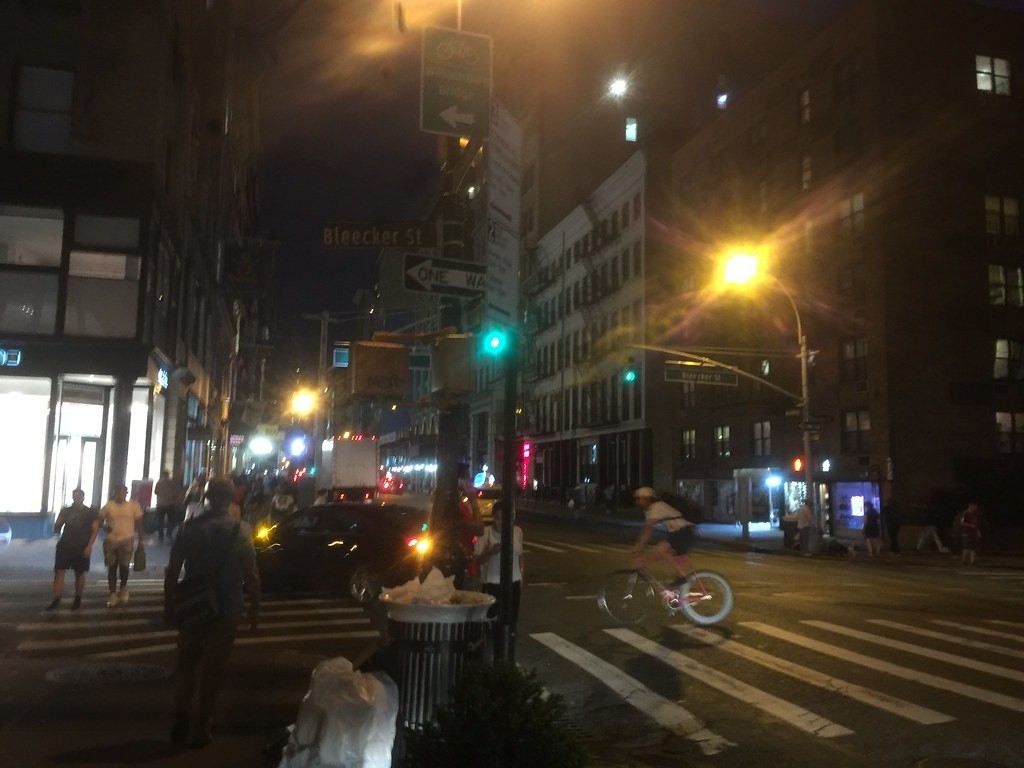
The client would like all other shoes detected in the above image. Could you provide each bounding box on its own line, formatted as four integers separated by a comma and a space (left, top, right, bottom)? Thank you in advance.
170, 716, 188, 744
191, 733, 213, 749
46, 601, 60, 610
120, 591, 129, 603
73, 600, 80, 609
109, 596, 118, 606
669, 578, 687, 588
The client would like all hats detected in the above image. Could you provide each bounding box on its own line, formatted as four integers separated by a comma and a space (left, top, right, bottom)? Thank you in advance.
203, 479, 241, 498
634, 486, 655, 498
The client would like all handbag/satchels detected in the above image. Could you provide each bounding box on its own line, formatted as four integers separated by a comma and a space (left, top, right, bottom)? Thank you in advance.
164, 574, 225, 632
134, 548, 146, 571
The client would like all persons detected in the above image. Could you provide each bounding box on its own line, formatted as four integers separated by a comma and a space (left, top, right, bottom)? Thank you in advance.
98, 484, 144, 607
914, 501, 947, 554
627, 487, 696, 603
131, 471, 178, 543
45, 486, 98, 610
457, 462, 524, 662
603, 484, 620, 516
229, 468, 300, 527
183, 478, 204, 523
163, 475, 264, 748
798, 499, 818, 557
314, 488, 328, 505
861, 501, 882, 560
882, 498, 901, 557
960, 500, 982, 560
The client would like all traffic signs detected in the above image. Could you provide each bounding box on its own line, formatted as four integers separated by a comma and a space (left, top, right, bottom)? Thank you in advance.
485, 218, 520, 329
419, 23, 493, 141
488, 95, 524, 236
402, 255, 490, 299
798, 421, 821, 441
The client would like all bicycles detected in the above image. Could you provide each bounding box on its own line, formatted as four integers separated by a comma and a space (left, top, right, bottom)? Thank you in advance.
596, 550, 735, 628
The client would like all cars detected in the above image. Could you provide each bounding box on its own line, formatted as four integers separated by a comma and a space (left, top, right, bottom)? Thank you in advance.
249, 502, 484, 591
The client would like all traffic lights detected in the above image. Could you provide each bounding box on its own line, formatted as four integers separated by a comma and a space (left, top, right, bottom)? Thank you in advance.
625, 352, 637, 383
486, 333, 505, 353
821, 459, 831, 473
429, 332, 479, 396
792, 458, 803, 473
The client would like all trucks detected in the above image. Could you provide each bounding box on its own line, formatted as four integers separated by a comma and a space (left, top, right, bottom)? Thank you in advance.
319, 436, 380, 503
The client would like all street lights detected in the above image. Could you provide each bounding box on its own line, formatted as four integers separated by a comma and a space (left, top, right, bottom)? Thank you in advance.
733, 254, 823, 553
